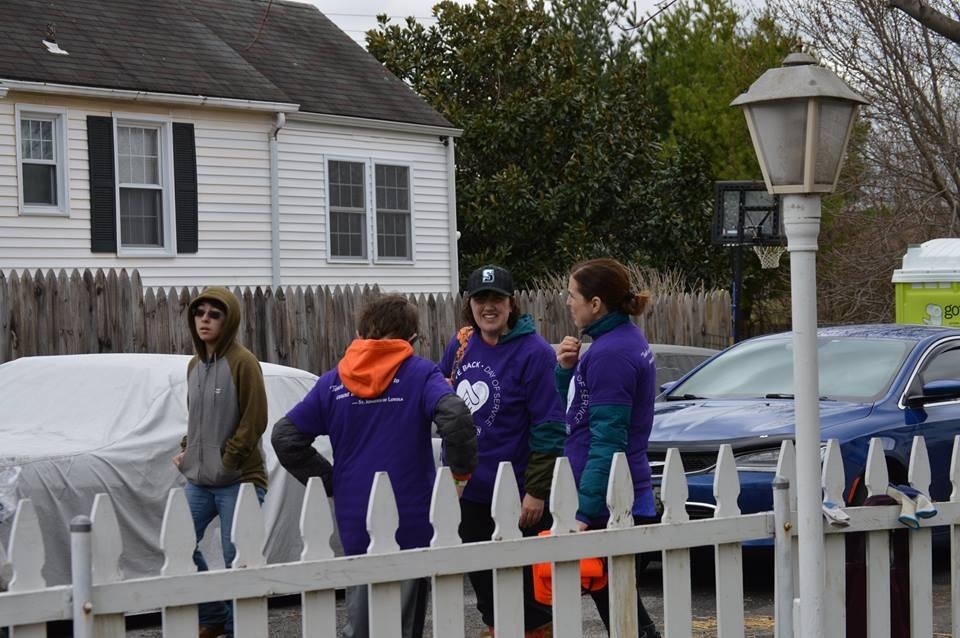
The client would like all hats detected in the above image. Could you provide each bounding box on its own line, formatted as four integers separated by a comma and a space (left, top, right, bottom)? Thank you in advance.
466, 264, 515, 296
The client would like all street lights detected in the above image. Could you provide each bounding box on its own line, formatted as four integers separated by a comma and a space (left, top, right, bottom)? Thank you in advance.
731, 48, 869, 638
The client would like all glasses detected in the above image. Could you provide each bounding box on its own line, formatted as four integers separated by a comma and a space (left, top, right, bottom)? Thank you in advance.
192, 308, 226, 320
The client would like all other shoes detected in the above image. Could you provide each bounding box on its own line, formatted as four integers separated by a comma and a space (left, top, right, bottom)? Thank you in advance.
198, 623, 226, 638
483, 621, 553, 638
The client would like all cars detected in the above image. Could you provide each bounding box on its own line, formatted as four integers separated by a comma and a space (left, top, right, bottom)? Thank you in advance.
0, 351, 339, 612
644, 323, 960, 593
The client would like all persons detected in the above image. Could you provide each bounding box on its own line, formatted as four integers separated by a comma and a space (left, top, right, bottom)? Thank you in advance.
172, 286, 271, 638
440, 258, 669, 638
267, 298, 478, 637
